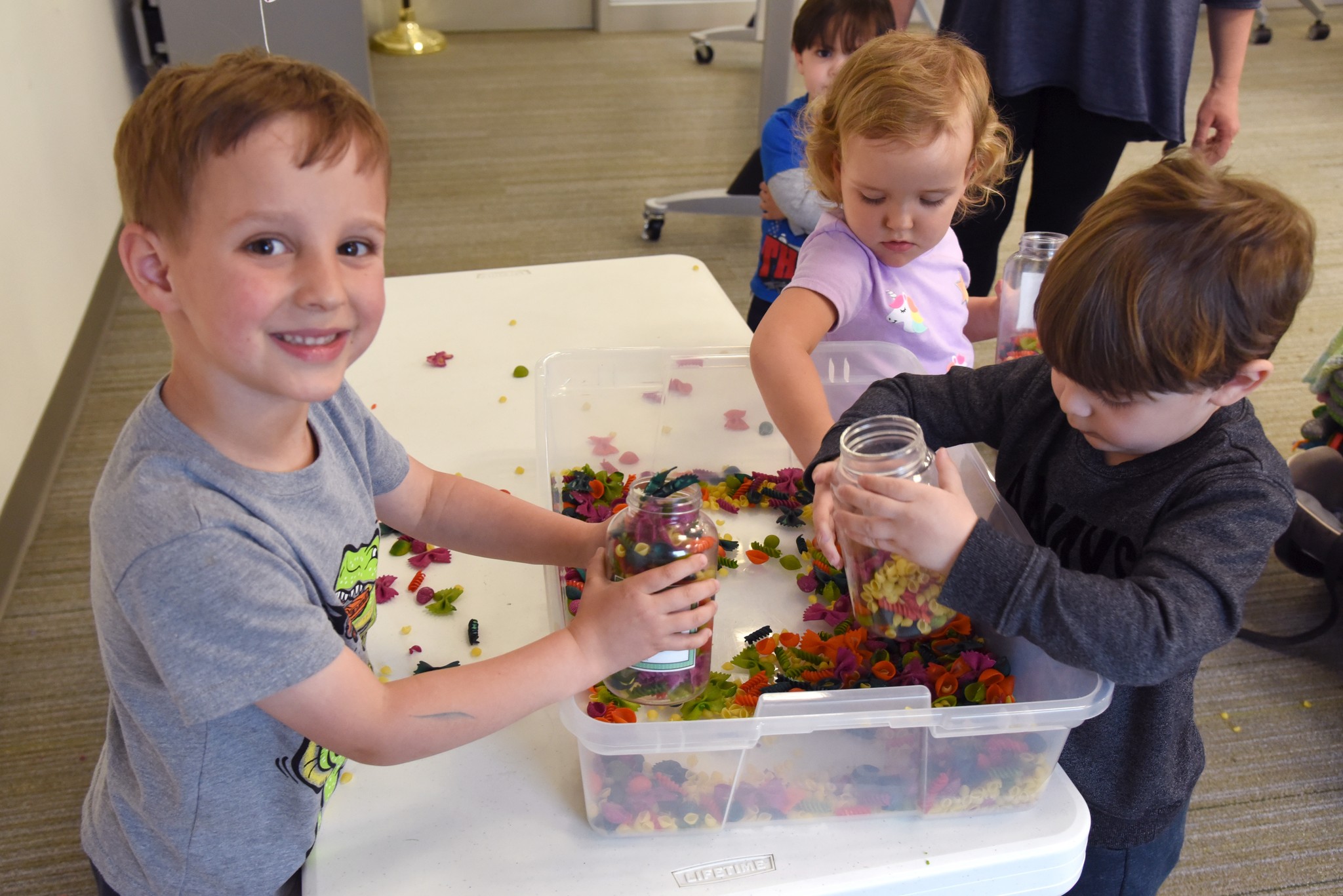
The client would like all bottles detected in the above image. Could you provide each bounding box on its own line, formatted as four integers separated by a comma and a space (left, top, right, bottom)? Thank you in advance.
601, 474, 719, 705
995, 230, 1068, 365
831, 414, 959, 641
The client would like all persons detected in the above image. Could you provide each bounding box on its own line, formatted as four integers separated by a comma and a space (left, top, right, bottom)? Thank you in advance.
934, 0, 1255, 296
793, 145, 1314, 896
80, 46, 719, 896
751, 0, 898, 340
749, 30, 1022, 605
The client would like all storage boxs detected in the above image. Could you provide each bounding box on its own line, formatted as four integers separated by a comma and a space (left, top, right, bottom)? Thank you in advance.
536, 342, 1114, 836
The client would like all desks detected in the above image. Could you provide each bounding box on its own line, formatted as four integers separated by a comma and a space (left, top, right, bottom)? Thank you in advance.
302, 253, 1090, 896
